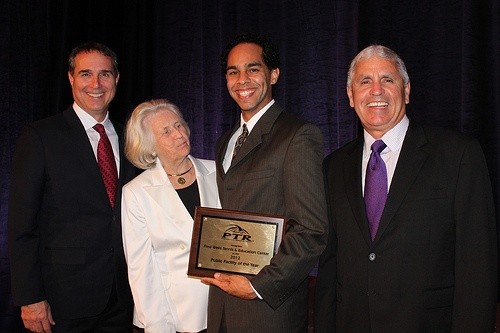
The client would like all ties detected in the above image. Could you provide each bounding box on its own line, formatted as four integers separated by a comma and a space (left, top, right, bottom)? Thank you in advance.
363, 139, 388, 240
231, 123, 249, 168
92, 123, 120, 209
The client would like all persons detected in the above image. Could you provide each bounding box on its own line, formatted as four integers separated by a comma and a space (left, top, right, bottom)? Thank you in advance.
200, 37, 331, 333
10, 42, 135, 332
121, 99, 225, 333
312, 46, 497, 332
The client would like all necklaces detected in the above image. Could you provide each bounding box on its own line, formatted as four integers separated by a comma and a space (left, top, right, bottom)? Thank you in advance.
166, 162, 195, 185
